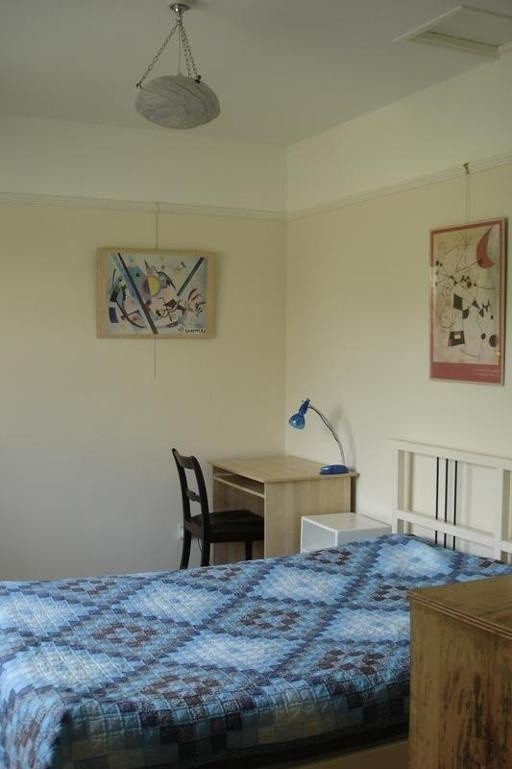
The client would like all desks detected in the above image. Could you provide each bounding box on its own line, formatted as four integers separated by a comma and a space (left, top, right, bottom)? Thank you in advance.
200, 450, 362, 565
399, 572, 510, 769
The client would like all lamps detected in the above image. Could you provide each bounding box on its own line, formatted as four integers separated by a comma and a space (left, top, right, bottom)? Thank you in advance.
129, 2, 219, 132
286, 396, 352, 475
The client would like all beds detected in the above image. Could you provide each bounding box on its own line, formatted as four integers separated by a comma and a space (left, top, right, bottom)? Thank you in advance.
0, 436, 511, 769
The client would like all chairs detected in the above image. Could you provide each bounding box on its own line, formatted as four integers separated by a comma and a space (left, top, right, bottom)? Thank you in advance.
167, 445, 264, 571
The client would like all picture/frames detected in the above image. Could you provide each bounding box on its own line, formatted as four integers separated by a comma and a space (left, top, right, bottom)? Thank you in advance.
93, 243, 218, 341
424, 215, 510, 388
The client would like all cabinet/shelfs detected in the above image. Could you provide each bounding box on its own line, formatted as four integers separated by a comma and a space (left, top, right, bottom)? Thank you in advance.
296, 511, 394, 556
